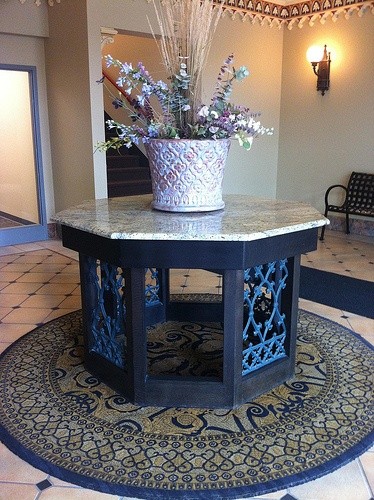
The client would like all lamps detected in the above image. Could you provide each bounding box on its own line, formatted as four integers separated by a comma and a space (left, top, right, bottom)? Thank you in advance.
306, 43, 333, 97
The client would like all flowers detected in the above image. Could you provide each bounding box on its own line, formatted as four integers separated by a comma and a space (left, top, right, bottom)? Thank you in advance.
92, 0, 275, 156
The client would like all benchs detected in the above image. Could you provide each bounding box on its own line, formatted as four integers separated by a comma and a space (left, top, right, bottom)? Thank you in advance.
319, 172, 374, 241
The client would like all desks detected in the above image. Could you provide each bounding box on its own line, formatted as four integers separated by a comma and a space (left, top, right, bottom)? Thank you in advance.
49, 193, 330, 409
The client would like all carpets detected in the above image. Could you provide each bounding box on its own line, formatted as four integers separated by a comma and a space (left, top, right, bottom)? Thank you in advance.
0, 293, 374, 500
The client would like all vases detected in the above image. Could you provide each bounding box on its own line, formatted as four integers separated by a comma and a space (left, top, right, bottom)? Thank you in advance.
143, 137, 232, 213
